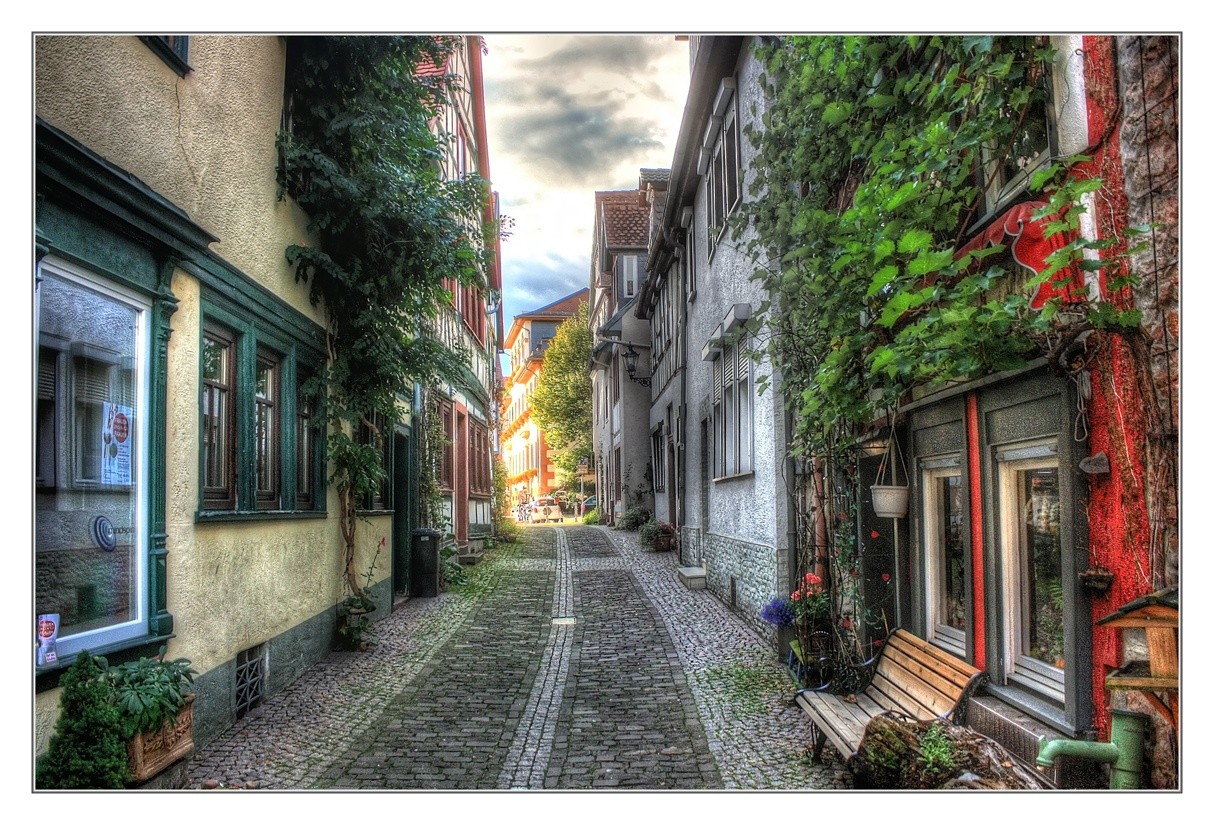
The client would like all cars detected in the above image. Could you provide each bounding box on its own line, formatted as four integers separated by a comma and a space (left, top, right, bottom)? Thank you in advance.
511, 496, 563, 523
581, 495, 597, 516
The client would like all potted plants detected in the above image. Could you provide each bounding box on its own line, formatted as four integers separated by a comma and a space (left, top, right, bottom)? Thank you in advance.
92, 643, 197, 781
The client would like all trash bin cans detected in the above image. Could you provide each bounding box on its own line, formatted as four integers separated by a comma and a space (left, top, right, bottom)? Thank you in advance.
413, 528, 445, 596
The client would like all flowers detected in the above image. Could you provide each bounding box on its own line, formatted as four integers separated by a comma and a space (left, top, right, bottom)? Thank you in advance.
760, 572, 832, 626
638, 515, 682, 553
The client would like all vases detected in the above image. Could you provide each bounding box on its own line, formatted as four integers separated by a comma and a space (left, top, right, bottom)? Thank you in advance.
869, 426, 913, 520
777, 623, 796, 664
653, 532, 671, 554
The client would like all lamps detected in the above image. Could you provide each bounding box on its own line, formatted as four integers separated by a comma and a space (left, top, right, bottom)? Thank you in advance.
622, 340, 652, 389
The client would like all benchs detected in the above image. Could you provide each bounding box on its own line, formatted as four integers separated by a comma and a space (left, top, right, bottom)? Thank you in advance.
793, 629, 983, 765
